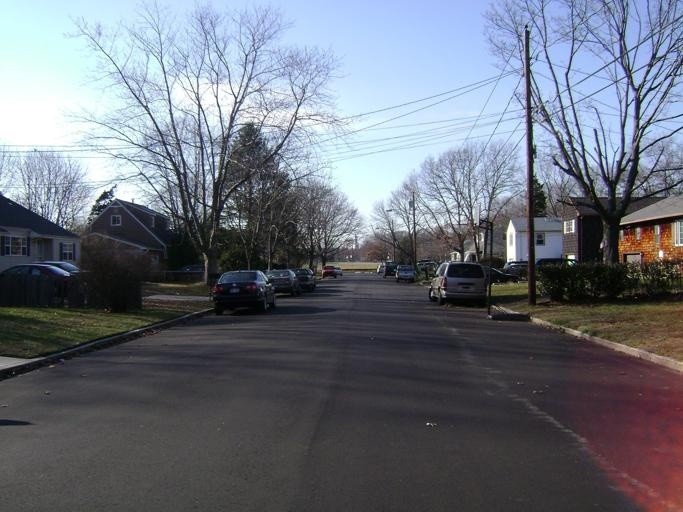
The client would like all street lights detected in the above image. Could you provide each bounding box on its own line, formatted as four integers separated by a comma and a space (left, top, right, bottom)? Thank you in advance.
384, 208, 415, 267
376, 228, 395, 262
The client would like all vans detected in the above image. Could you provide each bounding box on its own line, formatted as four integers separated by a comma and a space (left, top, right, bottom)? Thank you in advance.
428, 261, 487, 304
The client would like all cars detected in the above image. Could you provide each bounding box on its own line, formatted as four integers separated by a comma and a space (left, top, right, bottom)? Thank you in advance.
334, 266, 343, 276
0, 263, 72, 306
483, 258, 578, 284
320, 265, 337, 280
39, 261, 80, 276
211, 270, 277, 314
376, 258, 435, 282
293, 268, 317, 289
266, 269, 300, 296
